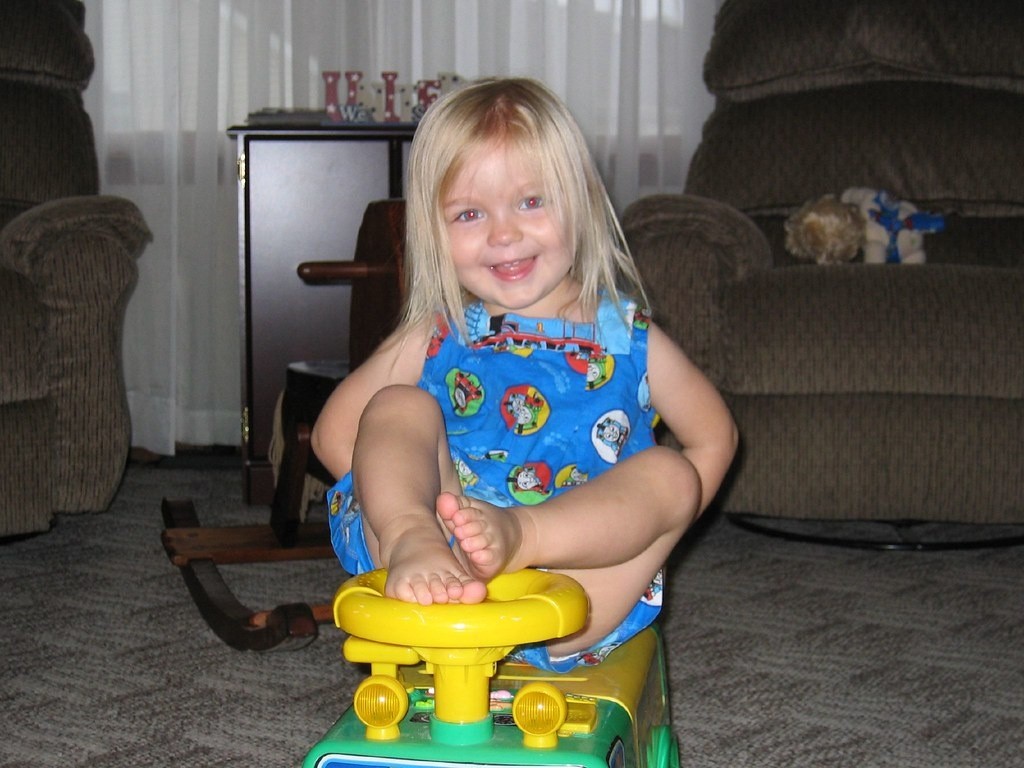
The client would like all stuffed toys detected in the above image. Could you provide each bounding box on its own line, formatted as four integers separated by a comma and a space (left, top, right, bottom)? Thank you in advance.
777, 188, 947, 265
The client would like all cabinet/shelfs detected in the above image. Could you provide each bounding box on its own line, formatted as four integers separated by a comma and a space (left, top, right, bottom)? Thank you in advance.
228, 121, 416, 468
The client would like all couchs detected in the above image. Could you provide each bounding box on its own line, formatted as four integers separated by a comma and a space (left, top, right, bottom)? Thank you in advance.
619, 0, 1024, 553
0, 0, 156, 538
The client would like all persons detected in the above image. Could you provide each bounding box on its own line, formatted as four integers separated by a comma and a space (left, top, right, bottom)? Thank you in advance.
312, 74, 739, 675
